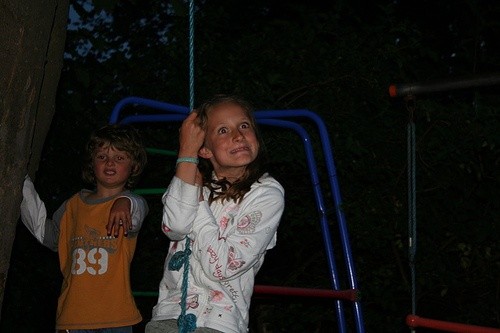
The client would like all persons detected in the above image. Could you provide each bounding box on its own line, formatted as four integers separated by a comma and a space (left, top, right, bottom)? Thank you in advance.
145, 95, 285, 333
19, 123, 148, 333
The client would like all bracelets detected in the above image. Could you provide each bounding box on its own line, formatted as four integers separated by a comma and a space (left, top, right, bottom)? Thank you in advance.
177, 157, 199, 165
